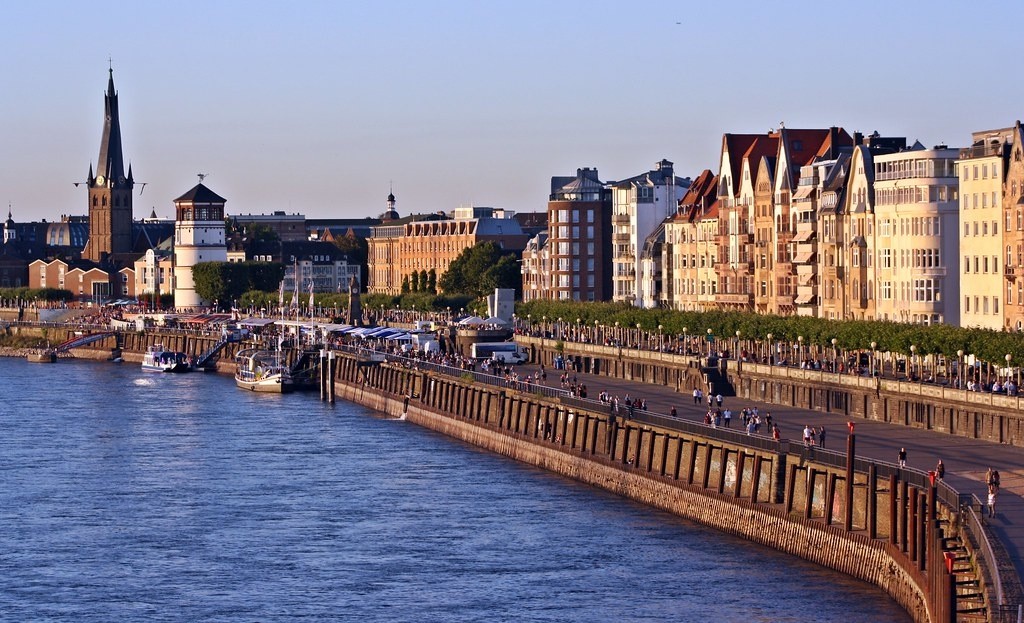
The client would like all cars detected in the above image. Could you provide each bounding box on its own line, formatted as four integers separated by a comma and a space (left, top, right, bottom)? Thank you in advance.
848, 352, 869, 367
935, 357, 956, 376
969, 360, 995, 377
896, 359, 909, 372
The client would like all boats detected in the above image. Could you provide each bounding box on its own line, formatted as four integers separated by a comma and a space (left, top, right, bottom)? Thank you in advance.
234, 347, 297, 394
27, 350, 52, 363
140, 343, 189, 373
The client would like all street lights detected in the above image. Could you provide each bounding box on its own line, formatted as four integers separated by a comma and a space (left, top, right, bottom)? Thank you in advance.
766, 333, 773, 365
215, 297, 479, 323
657, 324, 664, 361
1003, 354, 1012, 397
870, 341, 877, 379
576, 318, 582, 342
614, 320, 620, 351
910, 344, 917, 376
736, 330, 742, 359
706, 328, 712, 356
956, 349, 965, 389
526, 314, 533, 335
558, 317, 563, 340
682, 327, 688, 359
594, 319, 600, 345
638, 323, 642, 354
542, 315, 546, 339
797, 336, 804, 371
830, 338, 839, 374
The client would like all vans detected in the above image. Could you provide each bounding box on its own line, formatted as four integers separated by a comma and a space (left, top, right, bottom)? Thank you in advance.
490, 351, 525, 364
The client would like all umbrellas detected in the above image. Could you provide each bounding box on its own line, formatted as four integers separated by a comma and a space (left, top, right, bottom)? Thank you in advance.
459, 316, 507, 325
345, 317, 413, 343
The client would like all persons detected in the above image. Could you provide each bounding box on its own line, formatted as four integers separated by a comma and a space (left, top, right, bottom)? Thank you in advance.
936, 458, 945, 481
512, 325, 1024, 400
986, 489, 996, 519
985, 467, 1000, 494
164, 307, 213, 314
337, 337, 514, 377
31, 298, 163, 330
898, 447, 907, 468
624, 386, 827, 452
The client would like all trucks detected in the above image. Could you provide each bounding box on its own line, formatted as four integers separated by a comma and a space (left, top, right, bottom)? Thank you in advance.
470, 342, 530, 361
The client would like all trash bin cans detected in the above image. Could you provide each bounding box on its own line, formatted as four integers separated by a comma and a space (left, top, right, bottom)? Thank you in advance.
553, 358, 563, 370
575, 364, 582, 372
896, 360, 905, 371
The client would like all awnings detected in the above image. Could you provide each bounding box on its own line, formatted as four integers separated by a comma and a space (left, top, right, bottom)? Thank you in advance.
163, 314, 351, 331
792, 187, 814, 305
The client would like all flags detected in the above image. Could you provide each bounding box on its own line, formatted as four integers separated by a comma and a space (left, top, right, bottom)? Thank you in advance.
278, 280, 285, 309
290, 265, 305, 312
307, 281, 315, 310
